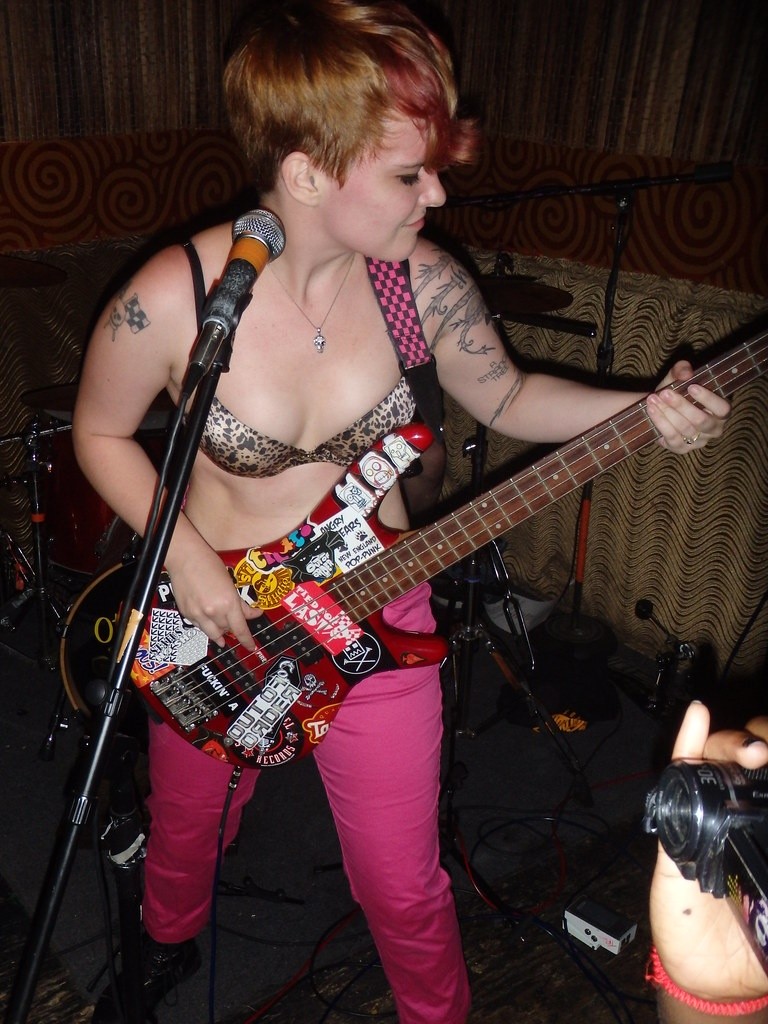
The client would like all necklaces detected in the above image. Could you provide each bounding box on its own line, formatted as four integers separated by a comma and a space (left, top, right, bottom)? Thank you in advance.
269, 256, 356, 354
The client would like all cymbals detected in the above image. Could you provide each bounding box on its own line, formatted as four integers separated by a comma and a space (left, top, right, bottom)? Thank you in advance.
22, 382, 176, 413
481, 275, 572, 316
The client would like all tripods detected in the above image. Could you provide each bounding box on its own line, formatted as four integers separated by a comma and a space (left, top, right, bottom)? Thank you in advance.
0, 424, 73, 671
439, 423, 598, 838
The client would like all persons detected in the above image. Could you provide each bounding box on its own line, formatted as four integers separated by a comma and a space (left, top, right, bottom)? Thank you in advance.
647, 699, 768, 1024
74, 0, 733, 1024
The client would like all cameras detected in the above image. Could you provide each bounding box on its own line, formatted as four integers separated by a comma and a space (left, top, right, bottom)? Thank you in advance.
645, 762, 768, 975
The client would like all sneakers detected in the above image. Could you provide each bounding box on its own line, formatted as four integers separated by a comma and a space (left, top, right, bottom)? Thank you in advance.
91, 938, 203, 1024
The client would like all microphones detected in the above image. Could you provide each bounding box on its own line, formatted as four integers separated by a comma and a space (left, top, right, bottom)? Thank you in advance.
183, 210, 286, 401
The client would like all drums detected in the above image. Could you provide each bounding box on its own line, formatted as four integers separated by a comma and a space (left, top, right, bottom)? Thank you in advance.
39, 405, 176, 577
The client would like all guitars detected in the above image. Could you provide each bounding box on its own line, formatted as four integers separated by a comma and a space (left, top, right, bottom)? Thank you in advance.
128, 328, 767, 768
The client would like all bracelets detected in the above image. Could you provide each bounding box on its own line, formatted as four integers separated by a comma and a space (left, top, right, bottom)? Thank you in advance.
645, 948, 768, 1016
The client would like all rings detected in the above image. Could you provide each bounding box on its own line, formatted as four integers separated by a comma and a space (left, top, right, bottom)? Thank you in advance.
684, 433, 700, 444
